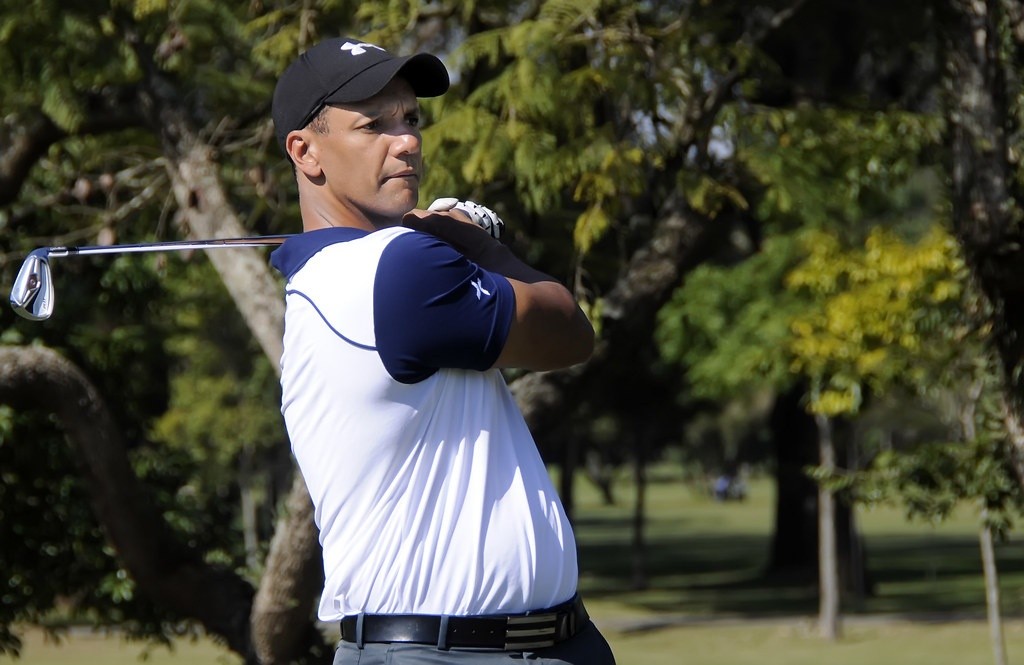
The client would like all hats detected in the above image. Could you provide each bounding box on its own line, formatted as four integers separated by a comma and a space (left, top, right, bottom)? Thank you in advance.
271, 38, 451, 156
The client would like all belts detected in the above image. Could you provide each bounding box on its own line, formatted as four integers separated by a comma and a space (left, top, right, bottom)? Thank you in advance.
339, 592, 585, 651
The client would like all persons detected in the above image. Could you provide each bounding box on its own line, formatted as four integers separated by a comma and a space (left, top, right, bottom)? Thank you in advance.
270, 38, 618, 665
715, 443, 748, 500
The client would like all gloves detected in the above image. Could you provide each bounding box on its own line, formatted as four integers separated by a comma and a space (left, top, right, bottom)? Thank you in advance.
425, 197, 506, 240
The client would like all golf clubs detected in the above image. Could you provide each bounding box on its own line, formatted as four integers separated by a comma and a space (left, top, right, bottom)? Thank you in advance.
8, 228, 305, 323
